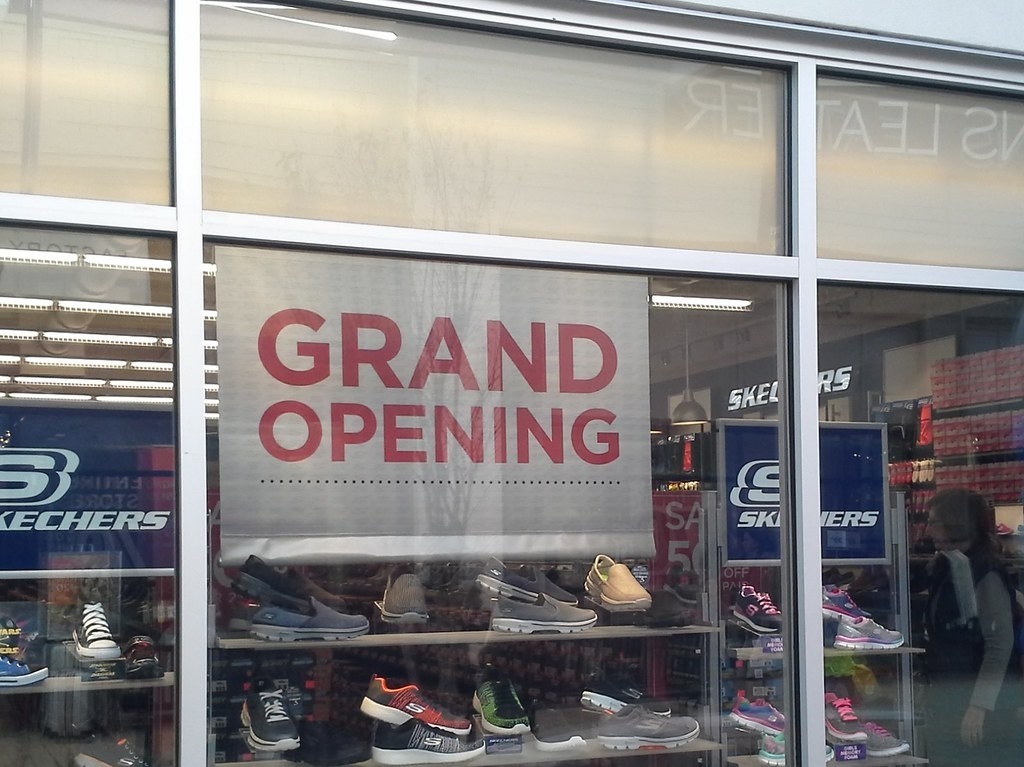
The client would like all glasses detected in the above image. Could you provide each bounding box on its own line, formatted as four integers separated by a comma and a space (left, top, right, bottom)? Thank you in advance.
927, 518, 943, 528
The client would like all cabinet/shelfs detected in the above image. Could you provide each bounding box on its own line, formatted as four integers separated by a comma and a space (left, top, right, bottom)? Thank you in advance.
0, 488, 933, 767
936, 397, 1024, 506
887, 481, 934, 558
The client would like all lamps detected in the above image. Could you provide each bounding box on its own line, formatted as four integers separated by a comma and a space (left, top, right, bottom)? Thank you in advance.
0, 249, 752, 422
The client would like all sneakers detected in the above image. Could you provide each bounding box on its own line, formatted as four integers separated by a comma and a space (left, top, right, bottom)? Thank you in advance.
822, 585, 873, 625
1017, 524, 1024, 534
859, 722, 910, 757
585, 554, 652, 611
227, 555, 369, 642
474, 556, 579, 607
360, 672, 472, 735
760, 733, 834, 766
825, 692, 868, 742
120, 635, 164, 678
74, 733, 150, 767
241, 675, 301, 752
597, 704, 700, 750
474, 677, 531, 735
0, 654, 49, 686
491, 592, 597, 634
733, 586, 782, 632
663, 566, 703, 603
381, 562, 427, 624
730, 691, 785, 735
580, 680, 671, 719
371, 718, 485, 765
834, 615, 904, 650
73, 601, 121, 659
993, 523, 1014, 535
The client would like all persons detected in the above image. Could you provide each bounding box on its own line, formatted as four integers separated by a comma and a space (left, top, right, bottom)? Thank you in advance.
917, 488, 1018, 767
